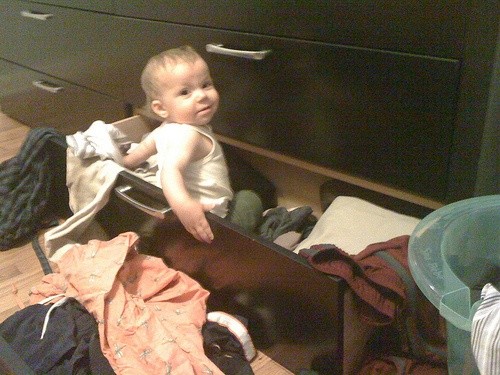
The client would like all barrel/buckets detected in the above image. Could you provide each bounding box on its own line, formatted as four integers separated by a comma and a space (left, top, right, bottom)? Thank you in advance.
407, 193, 500, 375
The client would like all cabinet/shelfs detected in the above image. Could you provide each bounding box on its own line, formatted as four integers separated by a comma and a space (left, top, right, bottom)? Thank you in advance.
0, 0, 500, 374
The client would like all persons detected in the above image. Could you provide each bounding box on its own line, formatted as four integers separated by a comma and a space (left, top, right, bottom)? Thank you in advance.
122, 46, 262, 244
